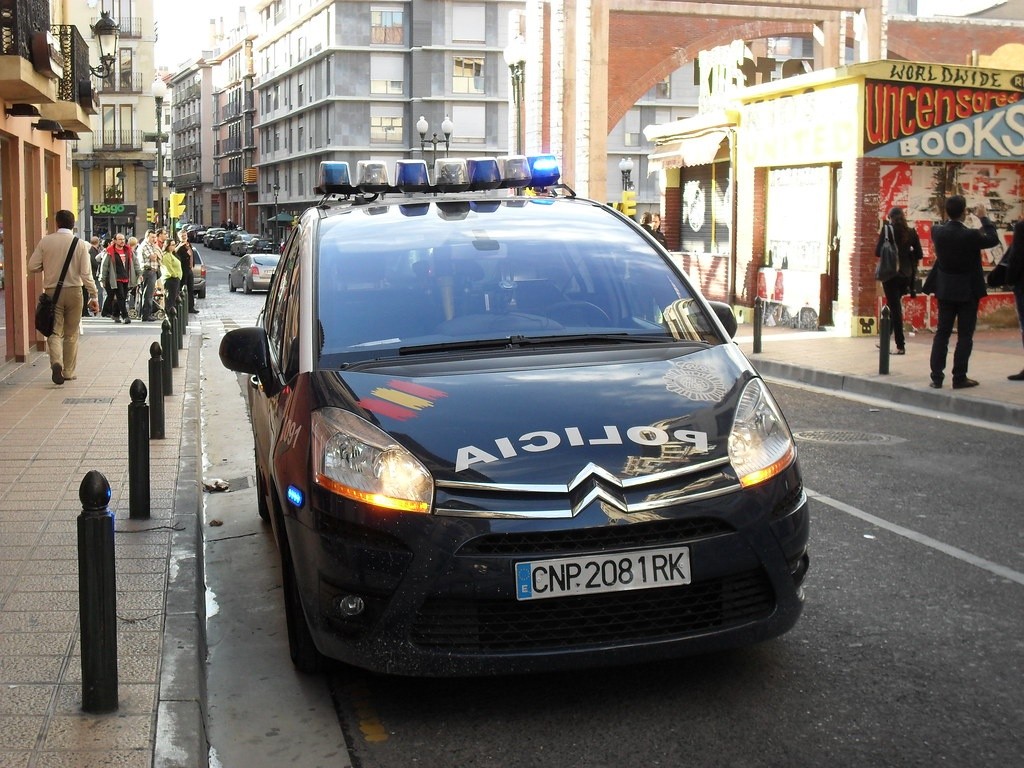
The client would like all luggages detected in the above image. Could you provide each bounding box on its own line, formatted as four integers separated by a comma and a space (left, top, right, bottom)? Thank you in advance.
127, 288, 138, 319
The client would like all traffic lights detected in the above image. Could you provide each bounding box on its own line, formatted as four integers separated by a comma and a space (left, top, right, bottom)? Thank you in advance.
146, 208, 155, 223
170, 193, 187, 218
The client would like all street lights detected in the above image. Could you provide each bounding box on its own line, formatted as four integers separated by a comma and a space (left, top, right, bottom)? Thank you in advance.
416, 115, 455, 169
272, 183, 283, 254
503, 34, 526, 196
192, 186, 197, 224
617, 157, 636, 192
152, 79, 167, 228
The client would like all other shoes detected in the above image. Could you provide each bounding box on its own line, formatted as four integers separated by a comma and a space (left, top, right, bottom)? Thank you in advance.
82, 309, 92, 317
189, 308, 199, 313
52, 363, 64, 384
930, 382, 942, 388
105, 312, 111, 317
890, 346, 905, 355
120, 315, 131, 323
111, 315, 121, 323
1008, 369, 1024, 381
953, 375, 978, 389
63, 376, 77, 380
142, 314, 158, 321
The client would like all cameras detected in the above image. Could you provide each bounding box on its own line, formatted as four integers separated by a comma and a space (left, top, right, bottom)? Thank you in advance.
966, 207, 976, 214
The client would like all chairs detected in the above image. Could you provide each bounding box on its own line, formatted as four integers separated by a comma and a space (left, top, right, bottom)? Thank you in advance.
323, 264, 419, 345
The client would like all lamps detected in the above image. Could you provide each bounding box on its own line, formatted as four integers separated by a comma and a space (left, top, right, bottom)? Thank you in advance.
5, 104, 41, 117
117, 172, 126, 186
31, 119, 64, 132
52, 130, 81, 143
89, 11, 121, 78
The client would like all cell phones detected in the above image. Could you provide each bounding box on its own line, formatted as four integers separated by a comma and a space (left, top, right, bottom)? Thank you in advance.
92, 301, 96, 316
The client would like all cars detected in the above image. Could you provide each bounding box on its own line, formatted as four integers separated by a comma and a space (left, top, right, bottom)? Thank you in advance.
182, 224, 214, 243
230, 234, 261, 257
228, 254, 282, 293
203, 227, 248, 251
218, 154, 811, 687
192, 245, 206, 298
246, 237, 279, 254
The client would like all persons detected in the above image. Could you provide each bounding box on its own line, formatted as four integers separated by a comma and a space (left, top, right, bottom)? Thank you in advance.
874, 208, 923, 354
638, 211, 669, 251
922, 195, 1000, 389
81, 228, 201, 323
1006, 219, 1024, 381
28, 210, 99, 384
221, 220, 237, 230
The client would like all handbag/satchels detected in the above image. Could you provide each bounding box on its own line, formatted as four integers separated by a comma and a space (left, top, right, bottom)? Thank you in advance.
875, 224, 900, 281
923, 273, 933, 295
907, 261, 922, 294
35, 293, 56, 337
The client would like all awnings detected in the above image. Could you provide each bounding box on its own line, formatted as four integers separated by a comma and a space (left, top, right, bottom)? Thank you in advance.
646, 132, 730, 178
